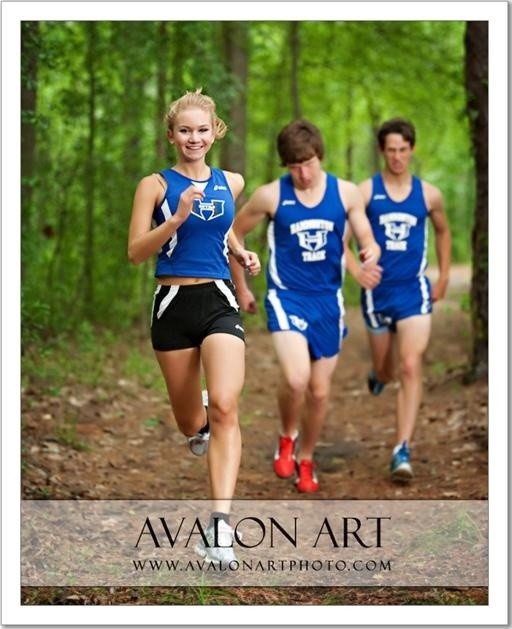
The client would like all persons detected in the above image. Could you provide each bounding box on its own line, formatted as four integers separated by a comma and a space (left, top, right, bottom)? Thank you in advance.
126, 87, 261, 565
342, 117, 451, 484
229, 119, 381, 493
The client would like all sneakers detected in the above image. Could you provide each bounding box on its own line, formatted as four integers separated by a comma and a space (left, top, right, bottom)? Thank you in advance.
194, 519, 242, 570
187, 389, 210, 456
389, 441, 413, 482
367, 367, 386, 397
289, 448, 321, 493
273, 427, 300, 479
